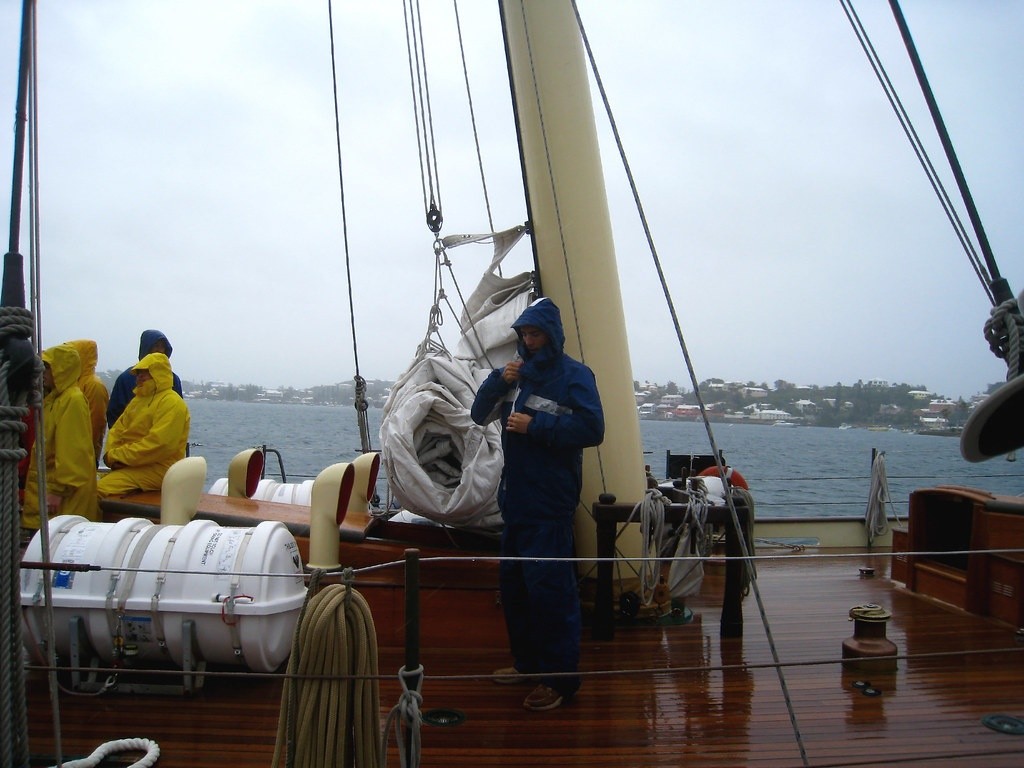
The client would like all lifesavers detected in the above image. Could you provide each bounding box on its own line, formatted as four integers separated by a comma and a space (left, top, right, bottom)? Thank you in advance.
697, 465, 748, 490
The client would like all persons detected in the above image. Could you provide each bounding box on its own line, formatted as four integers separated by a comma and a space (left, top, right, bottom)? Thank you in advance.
16, 329, 191, 539
471, 297, 606, 709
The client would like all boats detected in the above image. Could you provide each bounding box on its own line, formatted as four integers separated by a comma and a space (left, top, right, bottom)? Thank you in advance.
867, 427, 889, 432
772, 422, 798, 429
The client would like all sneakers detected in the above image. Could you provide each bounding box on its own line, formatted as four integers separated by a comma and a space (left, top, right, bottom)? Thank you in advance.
492, 667, 548, 683
524, 683, 579, 711
